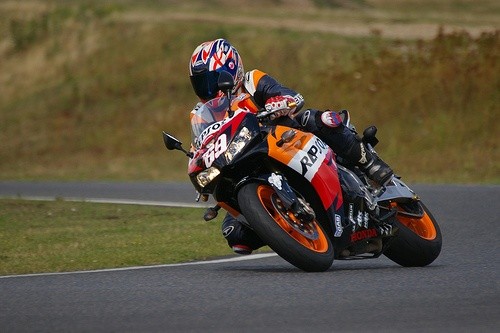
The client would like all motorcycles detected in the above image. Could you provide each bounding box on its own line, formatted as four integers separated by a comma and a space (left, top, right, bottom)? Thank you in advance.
162, 70, 443, 272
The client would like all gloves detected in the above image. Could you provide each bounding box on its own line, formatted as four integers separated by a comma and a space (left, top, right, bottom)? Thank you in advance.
265, 95, 296, 120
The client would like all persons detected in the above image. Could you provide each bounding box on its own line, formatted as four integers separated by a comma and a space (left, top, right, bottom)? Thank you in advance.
189, 39, 395, 254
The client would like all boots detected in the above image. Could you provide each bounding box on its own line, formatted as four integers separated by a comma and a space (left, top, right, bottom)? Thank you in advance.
345, 138, 393, 184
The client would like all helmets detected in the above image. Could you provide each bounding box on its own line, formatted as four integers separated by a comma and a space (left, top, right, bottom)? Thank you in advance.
189, 38, 245, 111
